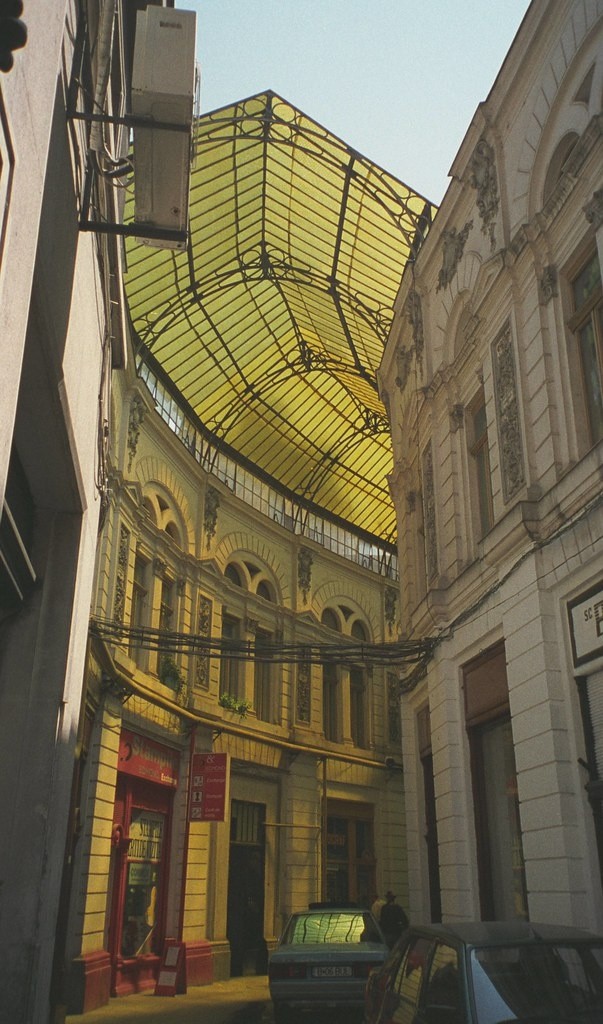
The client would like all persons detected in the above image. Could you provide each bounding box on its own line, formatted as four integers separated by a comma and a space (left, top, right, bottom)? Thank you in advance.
379, 891, 409, 951
372, 891, 387, 921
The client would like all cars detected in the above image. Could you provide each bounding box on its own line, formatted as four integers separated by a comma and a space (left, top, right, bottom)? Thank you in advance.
364, 920, 603, 1024
267, 908, 390, 1024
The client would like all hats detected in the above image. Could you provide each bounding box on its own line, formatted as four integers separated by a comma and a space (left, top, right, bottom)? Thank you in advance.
386, 891, 396, 897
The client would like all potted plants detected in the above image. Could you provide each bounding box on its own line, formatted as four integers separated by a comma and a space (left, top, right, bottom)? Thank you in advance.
162, 655, 185, 696
222, 693, 252, 722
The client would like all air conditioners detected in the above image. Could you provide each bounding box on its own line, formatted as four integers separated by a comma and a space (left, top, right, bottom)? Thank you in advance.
132, 4, 197, 252
